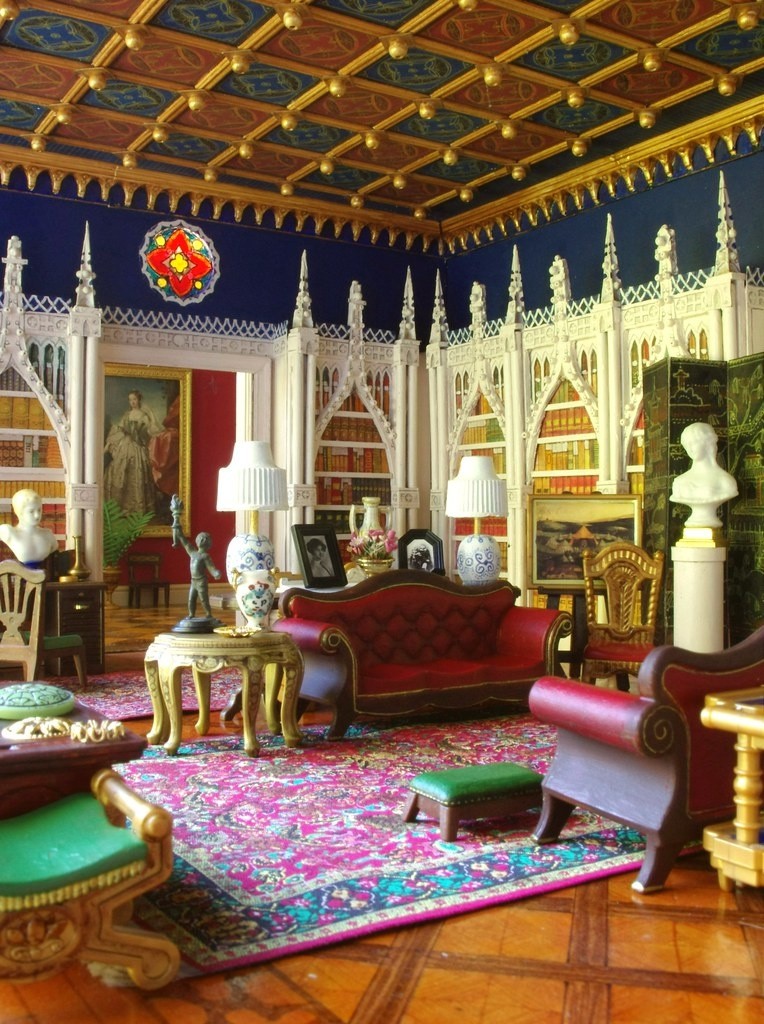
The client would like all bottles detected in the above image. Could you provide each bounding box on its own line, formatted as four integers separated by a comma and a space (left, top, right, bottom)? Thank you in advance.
68, 536, 92, 579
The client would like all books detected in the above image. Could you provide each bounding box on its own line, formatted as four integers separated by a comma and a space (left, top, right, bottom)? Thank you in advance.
314, 511, 386, 535
315, 477, 391, 505
314, 415, 389, 443
0, 505, 66, 533
316, 367, 390, 415
455, 518, 506, 574
338, 540, 358, 565
533, 350, 599, 438
453, 365, 508, 474
453, 518, 508, 585
0, 345, 64, 396
626, 400, 646, 510
532, 475, 599, 495
626, 328, 709, 386
315, 445, 390, 473
0, 482, 65, 499
0, 434, 63, 467
532, 440, 600, 471
1, 396, 63, 430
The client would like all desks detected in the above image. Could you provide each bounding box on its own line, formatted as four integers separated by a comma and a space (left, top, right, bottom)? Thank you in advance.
0, 676, 150, 819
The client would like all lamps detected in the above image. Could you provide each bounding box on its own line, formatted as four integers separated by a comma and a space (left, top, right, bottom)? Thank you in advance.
213, 431, 292, 588
441, 453, 512, 587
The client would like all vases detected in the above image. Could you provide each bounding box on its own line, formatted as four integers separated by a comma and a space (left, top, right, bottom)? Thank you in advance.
232, 566, 278, 630
66, 536, 94, 580
356, 558, 396, 580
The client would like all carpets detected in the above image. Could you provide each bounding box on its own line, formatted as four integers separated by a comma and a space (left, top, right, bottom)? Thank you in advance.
0, 664, 262, 723
90, 714, 716, 995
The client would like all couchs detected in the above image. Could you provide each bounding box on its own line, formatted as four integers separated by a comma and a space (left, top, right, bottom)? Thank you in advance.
270, 567, 574, 746
527, 594, 764, 898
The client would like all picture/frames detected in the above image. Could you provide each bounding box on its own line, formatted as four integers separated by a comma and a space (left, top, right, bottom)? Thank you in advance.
290, 522, 349, 588
104, 363, 194, 539
521, 491, 645, 588
397, 525, 447, 575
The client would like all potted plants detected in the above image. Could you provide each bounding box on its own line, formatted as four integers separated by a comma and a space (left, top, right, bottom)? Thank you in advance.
104, 495, 153, 612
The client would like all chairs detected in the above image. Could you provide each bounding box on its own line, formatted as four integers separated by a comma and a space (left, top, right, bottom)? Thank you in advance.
0, 557, 89, 691
578, 543, 668, 687
124, 552, 171, 608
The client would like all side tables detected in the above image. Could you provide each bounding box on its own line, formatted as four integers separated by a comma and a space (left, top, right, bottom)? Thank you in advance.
141, 629, 307, 760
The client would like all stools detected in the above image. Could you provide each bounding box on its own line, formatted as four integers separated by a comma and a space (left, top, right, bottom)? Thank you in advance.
397, 760, 544, 840
0, 766, 186, 994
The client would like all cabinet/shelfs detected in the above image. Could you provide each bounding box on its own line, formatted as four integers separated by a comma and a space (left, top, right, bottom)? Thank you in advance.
0, 575, 113, 680
273, 336, 403, 557
621, 283, 724, 487
0, 322, 87, 566
524, 310, 609, 494
426, 326, 518, 584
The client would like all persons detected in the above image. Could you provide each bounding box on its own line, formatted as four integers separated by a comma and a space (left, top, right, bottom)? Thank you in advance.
412, 545, 434, 571
307, 537, 335, 577
172, 524, 222, 618
0, 488, 59, 562
675, 423, 738, 498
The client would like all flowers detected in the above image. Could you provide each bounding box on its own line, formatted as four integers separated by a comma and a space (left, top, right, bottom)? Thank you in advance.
346, 527, 400, 555
